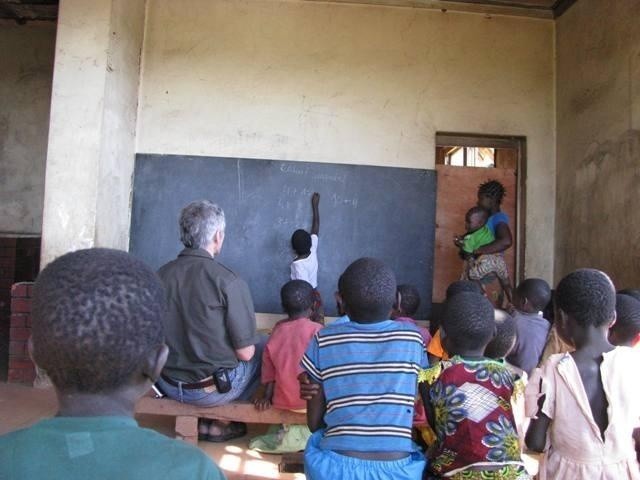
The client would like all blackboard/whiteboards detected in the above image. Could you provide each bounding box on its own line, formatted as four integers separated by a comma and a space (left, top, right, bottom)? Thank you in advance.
135, 153, 436, 320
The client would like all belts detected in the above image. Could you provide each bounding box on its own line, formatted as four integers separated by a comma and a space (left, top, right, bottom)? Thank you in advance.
160, 373, 215, 390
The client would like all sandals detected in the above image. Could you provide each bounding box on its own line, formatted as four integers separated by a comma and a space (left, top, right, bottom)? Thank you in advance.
209, 421, 247, 442
198, 421, 211, 440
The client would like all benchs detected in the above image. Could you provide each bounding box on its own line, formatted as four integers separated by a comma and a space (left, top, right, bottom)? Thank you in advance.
141, 396, 312, 444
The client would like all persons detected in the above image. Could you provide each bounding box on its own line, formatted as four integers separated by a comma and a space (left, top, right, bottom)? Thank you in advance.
0, 247, 227, 479
459, 205, 513, 303
453, 178, 513, 304
252, 279, 325, 414
391, 268, 639, 480
290, 191, 320, 289
152, 197, 269, 442
297, 257, 429, 480
